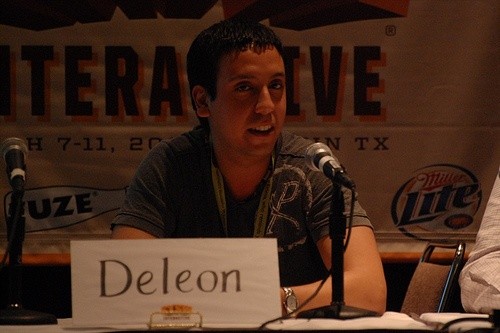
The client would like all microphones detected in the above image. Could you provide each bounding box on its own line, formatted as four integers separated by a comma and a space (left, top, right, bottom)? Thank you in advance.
0, 137, 29, 191
304, 142, 356, 190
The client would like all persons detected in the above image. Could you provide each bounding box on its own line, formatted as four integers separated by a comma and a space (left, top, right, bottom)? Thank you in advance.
458, 165, 500, 316
109, 16, 387, 320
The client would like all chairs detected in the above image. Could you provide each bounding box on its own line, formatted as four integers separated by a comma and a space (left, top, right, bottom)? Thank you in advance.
401, 240, 466, 315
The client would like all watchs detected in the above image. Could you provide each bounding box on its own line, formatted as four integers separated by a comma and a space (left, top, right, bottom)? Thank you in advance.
281, 287, 299, 319
